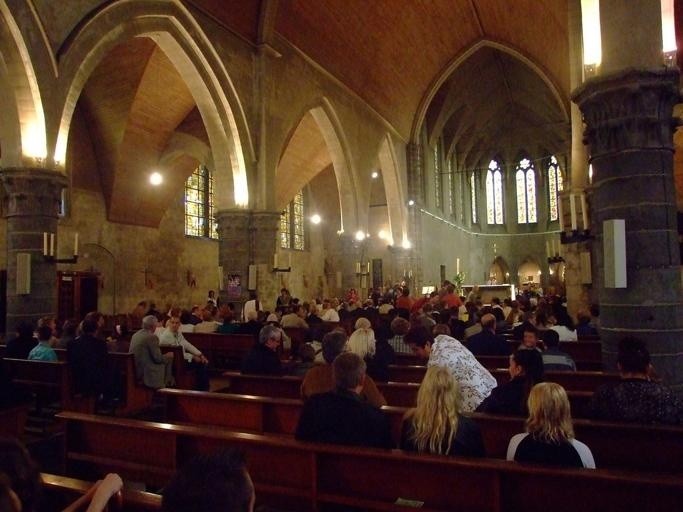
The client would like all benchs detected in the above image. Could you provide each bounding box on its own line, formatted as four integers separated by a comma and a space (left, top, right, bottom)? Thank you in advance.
0, 298, 682, 511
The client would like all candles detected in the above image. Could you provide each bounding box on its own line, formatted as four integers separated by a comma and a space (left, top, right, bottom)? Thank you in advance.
354, 262, 370, 273
402, 269, 413, 277
42, 231, 79, 257
272, 251, 292, 267
545, 192, 588, 257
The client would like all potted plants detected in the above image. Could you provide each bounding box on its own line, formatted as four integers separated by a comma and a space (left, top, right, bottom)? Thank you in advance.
453, 271, 465, 296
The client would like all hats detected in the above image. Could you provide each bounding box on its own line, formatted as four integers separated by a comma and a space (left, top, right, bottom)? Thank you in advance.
267, 313, 278, 321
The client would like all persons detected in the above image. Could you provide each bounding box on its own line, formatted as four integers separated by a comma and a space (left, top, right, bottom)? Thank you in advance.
586, 336, 682, 428
3, 290, 242, 408
241, 281, 599, 416
0, 437, 122, 512
503, 381, 596, 469
399, 363, 485, 459
161, 456, 255, 512
293, 351, 392, 449
